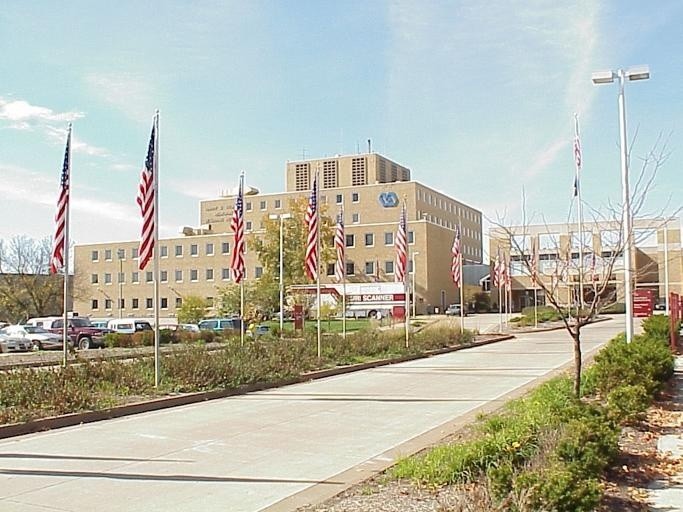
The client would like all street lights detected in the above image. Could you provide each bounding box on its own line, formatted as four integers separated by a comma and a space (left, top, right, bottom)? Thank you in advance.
587, 66, 652, 342
269, 212, 296, 340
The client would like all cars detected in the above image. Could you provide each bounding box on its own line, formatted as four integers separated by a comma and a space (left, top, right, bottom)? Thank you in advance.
444, 302, 469, 317
654, 298, 666, 310
0, 310, 251, 356
254, 323, 273, 340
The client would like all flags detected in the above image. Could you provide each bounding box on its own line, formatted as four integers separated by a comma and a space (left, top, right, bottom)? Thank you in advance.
228, 178, 245, 285
301, 174, 319, 284
392, 204, 406, 283
50, 134, 70, 274
135, 116, 156, 269
500, 256, 504, 289
571, 133, 581, 196
530, 242, 537, 287
504, 259, 510, 290
332, 206, 343, 282
492, 249, 499, 290
450, 227, 460, 289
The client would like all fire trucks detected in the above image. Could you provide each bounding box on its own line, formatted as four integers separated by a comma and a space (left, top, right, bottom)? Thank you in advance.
281, 279, 412, 322
